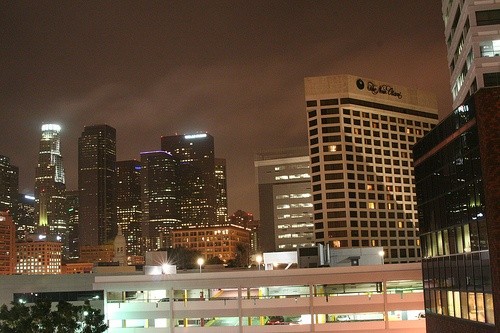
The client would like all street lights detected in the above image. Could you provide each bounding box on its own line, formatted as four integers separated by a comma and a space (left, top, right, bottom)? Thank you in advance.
255, 256, 261, 270
196, 258, 205, 273
